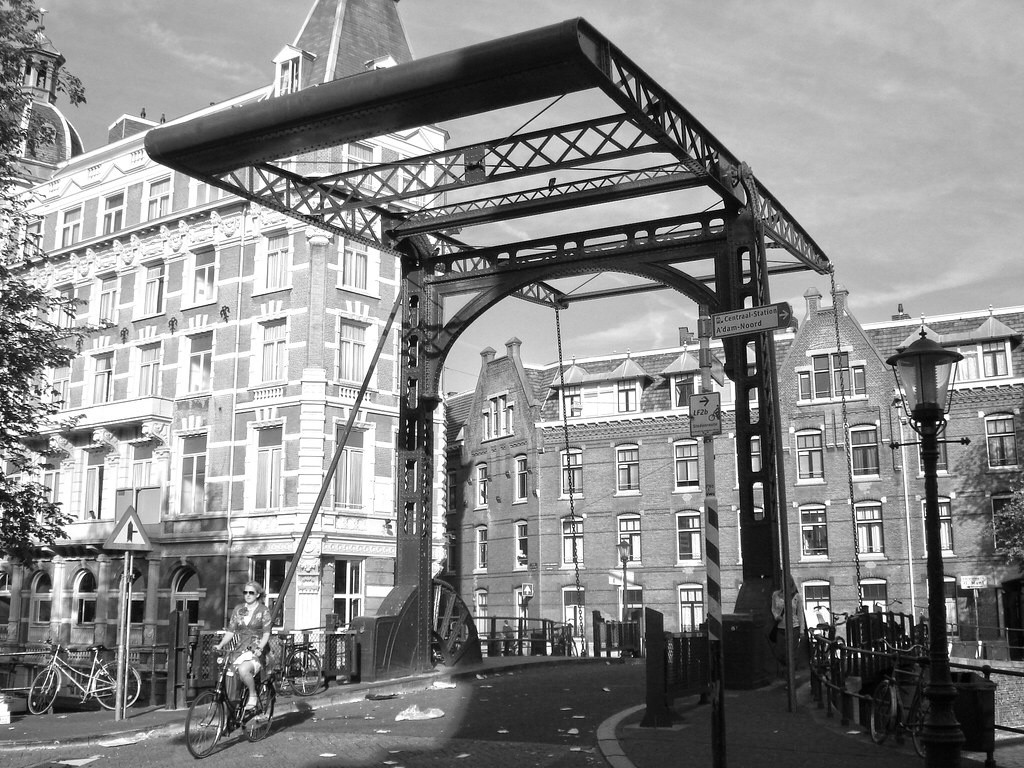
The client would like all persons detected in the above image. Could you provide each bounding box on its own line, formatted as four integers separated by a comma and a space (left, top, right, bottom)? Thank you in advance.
211, 581, 276, 736
502, 620, 514, 638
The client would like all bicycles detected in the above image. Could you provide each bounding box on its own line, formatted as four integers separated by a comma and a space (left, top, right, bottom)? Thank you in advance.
875, 600, 902, 637
272, 635, 322, 695
553, 618, 579, 658
808, 606, 849, 689
27, 638, 142, 715
185, 646, 277, 759
870, 637, 933, 758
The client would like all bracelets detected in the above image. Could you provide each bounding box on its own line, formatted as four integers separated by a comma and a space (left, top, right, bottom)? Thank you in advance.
256, 647, 264, 652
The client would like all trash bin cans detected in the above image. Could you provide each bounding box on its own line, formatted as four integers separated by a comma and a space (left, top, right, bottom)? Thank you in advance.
951, 670, 996, 753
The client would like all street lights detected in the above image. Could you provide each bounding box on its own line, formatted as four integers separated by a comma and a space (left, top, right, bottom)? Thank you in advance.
885, 326, 972, 768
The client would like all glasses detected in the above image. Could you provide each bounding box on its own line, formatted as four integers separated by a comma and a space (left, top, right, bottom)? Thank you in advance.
243, 591, 255, 595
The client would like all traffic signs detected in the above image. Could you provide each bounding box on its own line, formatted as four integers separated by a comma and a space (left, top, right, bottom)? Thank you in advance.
711, 301, 793, 340
689, 392, 722, 437
